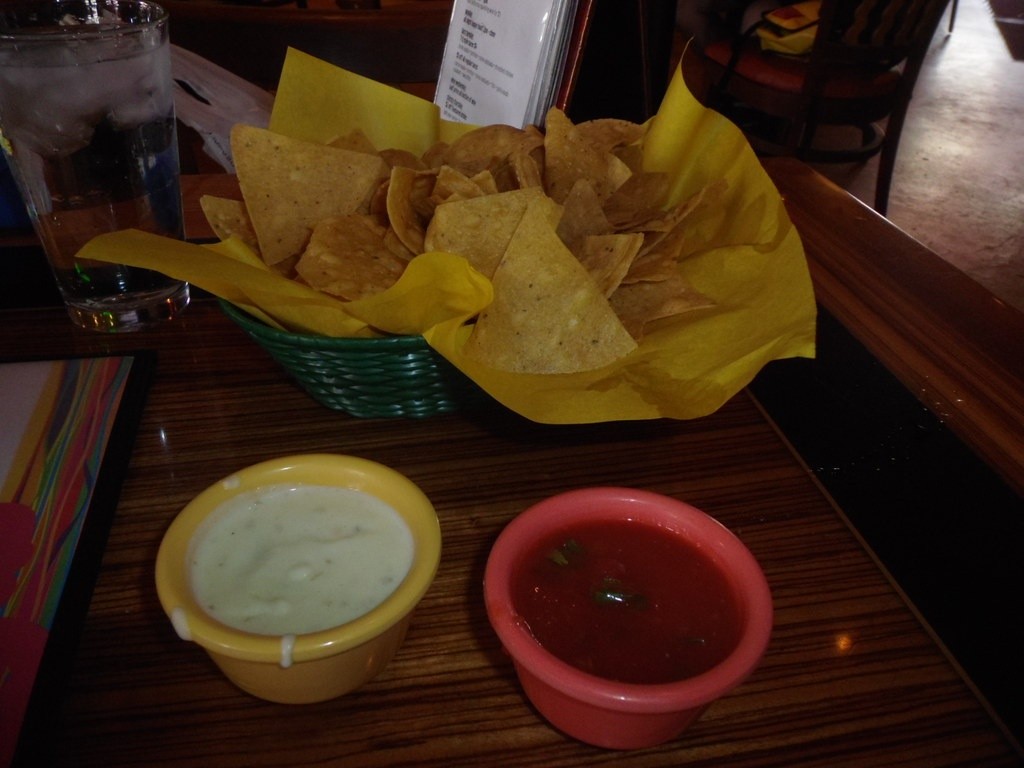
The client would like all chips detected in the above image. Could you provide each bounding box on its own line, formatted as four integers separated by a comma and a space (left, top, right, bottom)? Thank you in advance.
198, 106, 711, 376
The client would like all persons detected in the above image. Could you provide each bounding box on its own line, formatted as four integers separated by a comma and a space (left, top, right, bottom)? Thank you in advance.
676, 0, 755, 64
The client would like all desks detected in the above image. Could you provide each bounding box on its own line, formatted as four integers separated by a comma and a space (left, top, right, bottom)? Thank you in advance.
0, 150, 1024, 768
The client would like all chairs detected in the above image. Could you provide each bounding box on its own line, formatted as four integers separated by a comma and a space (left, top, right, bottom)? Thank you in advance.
701, 0, 951, 218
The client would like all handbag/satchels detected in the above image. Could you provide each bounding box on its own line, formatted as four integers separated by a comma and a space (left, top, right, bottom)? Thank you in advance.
753, 0, 821, 56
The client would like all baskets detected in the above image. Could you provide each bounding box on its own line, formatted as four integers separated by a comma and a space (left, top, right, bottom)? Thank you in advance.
219, 286, 481, 420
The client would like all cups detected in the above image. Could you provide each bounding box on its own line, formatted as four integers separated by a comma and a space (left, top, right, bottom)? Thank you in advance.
0, 0, 191, 335
483, 486, 774, 749
154, 453, 442, 705
